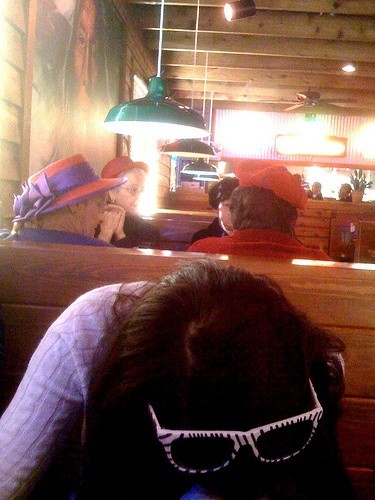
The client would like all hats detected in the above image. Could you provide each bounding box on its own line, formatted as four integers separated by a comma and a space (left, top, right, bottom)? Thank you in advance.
10, 155, 126, 222
209, 176, 237, 209
102, 155, 149, 180
233, 157, 308, 209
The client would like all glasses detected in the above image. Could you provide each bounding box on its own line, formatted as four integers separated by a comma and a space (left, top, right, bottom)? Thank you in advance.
146, 373, 324, 474
118, 184, 145, 196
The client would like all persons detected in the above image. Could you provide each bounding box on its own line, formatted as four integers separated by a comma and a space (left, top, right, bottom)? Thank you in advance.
192, 177, 239, 242
338, 183, 352, 202
187, 159, 332, 260
0, 259, 351, 500
0, 153, 128, 248
307, 182, 323, 200
94, 157, 161, 249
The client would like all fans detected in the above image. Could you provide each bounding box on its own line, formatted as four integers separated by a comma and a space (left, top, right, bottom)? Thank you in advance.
258, 87, 357, 112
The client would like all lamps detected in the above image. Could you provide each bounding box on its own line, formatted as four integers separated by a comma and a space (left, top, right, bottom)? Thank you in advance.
224, 0, 256, 22
104, 0, 219, 181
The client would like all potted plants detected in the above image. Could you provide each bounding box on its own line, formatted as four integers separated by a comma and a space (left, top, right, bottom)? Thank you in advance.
338, 223, 358, 259
349, 168, 373, 202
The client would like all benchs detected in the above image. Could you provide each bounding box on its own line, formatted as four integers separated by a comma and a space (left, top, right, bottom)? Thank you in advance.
0, 193, 375, 500
